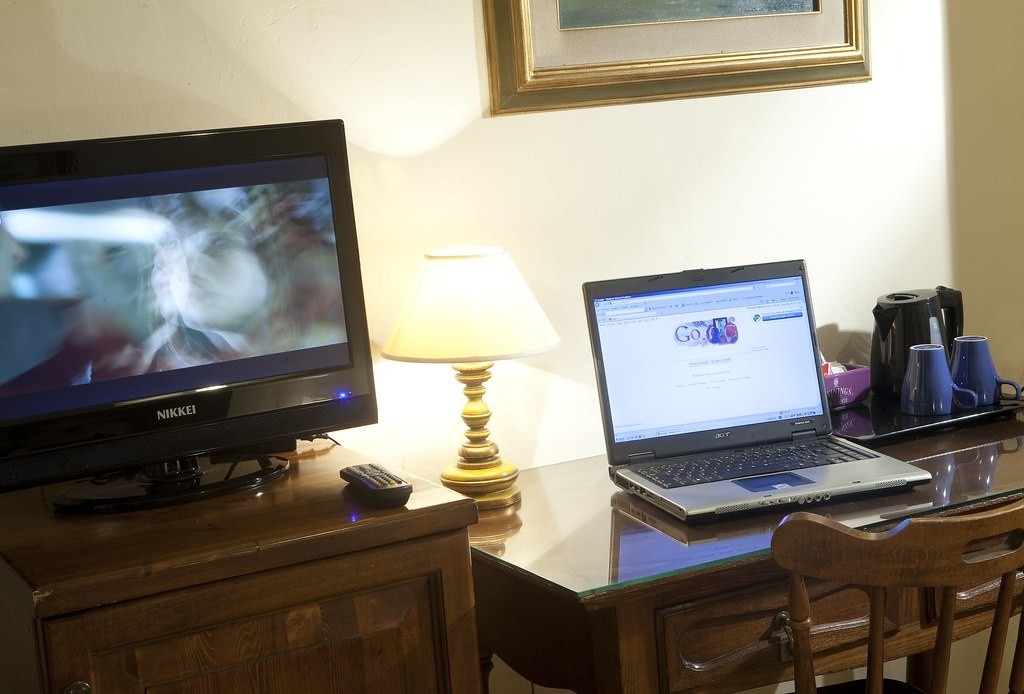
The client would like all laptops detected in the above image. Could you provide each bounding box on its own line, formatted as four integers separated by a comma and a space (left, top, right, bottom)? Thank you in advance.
583, 259, 933, 525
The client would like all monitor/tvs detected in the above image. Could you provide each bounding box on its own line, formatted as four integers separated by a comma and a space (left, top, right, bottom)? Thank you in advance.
0, 117, 377, 514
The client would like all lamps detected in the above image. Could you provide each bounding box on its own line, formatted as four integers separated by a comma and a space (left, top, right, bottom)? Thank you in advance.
376, 246, 560, 511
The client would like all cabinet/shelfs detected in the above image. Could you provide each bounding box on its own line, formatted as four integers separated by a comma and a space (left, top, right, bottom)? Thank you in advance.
0, 433, 479, 694
468, 408, 1024, 694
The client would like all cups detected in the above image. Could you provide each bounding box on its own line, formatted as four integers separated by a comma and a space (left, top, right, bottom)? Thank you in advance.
955, 436, 1023, 496
915, 454, 961, 507
900, 343, 978, 416
950, 335, 1022, 406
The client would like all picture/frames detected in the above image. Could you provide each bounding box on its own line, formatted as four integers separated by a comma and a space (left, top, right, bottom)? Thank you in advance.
482, 0, 871, 117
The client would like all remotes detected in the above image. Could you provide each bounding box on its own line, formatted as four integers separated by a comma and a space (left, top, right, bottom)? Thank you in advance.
339, 461, 413, 508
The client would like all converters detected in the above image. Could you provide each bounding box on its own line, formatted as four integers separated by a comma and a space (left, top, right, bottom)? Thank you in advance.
210, 438, 297, 455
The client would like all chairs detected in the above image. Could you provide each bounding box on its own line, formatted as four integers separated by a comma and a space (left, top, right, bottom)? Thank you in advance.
771, 498, 1024, 694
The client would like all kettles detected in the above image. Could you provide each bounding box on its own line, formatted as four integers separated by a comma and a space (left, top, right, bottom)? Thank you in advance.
869, 280, 964, 407
